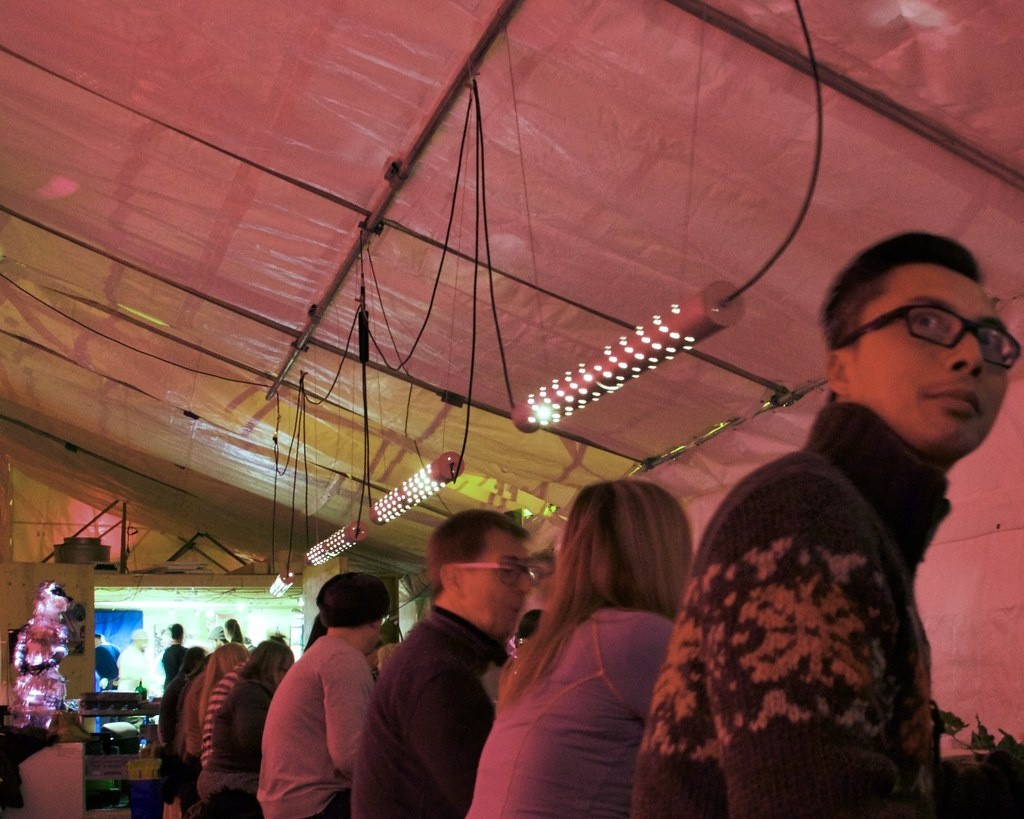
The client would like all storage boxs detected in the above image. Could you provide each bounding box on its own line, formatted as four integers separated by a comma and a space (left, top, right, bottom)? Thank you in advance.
84, 749, 164, 819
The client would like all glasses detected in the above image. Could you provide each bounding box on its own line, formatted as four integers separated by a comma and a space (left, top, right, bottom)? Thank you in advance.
839, 303, 1021, 369
450, 562, 547, 588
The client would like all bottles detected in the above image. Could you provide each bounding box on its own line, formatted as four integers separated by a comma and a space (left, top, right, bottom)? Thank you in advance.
135, 682, 147, 703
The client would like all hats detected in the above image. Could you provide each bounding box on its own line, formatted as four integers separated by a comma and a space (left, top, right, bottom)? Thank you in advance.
316, 571, 390, 628
132, 630, 149, 639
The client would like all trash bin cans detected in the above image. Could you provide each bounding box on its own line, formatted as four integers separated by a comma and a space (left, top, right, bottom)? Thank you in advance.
101, 721, 141, 754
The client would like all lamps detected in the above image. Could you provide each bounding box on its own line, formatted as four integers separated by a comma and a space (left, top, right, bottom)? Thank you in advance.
270, 569, 295, 597
367, 452, 465, 525
305, 519, 370, 568
510, 282, 747, 432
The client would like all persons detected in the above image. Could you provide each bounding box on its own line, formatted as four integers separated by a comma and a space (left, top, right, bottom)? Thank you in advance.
94, 230, 1022, 819
